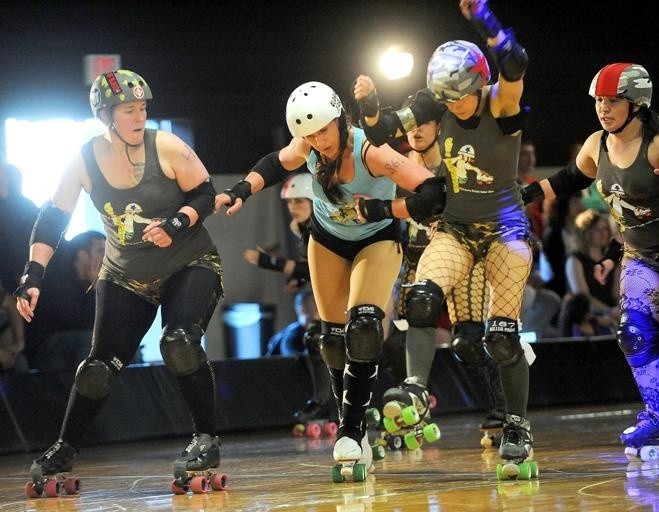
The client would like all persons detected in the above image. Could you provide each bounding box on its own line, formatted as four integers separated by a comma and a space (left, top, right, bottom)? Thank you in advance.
13, 69, 226, 497
265, 289, 319, 355
350, 1, 541, 479
32, 231, 146, 372
212, 81, 448, 482
0, 164, 42, 292
519, 137, 621, 335
520, 61, 659, 461
243, 172, 341, 438
0, 288, 30, 371
394, 95, 507, 448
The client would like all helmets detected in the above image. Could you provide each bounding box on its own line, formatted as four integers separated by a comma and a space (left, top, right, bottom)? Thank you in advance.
285, 81, 345, 138
90, 70, 154, 119
588, 63, 653, 108
426, 40, 492, 104
281, 173, 314, 201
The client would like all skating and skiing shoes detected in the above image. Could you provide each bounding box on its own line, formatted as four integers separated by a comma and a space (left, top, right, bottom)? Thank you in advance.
381, 376, 440, 450
331, 416, 372, 483
171, 433, 229, 494
363, 408, 403, 459
25, 439, 81, 498
291, 399, 337, 436
496, 413, 539, 479
619, 406, 659, 462
479, 409, 505, 449
